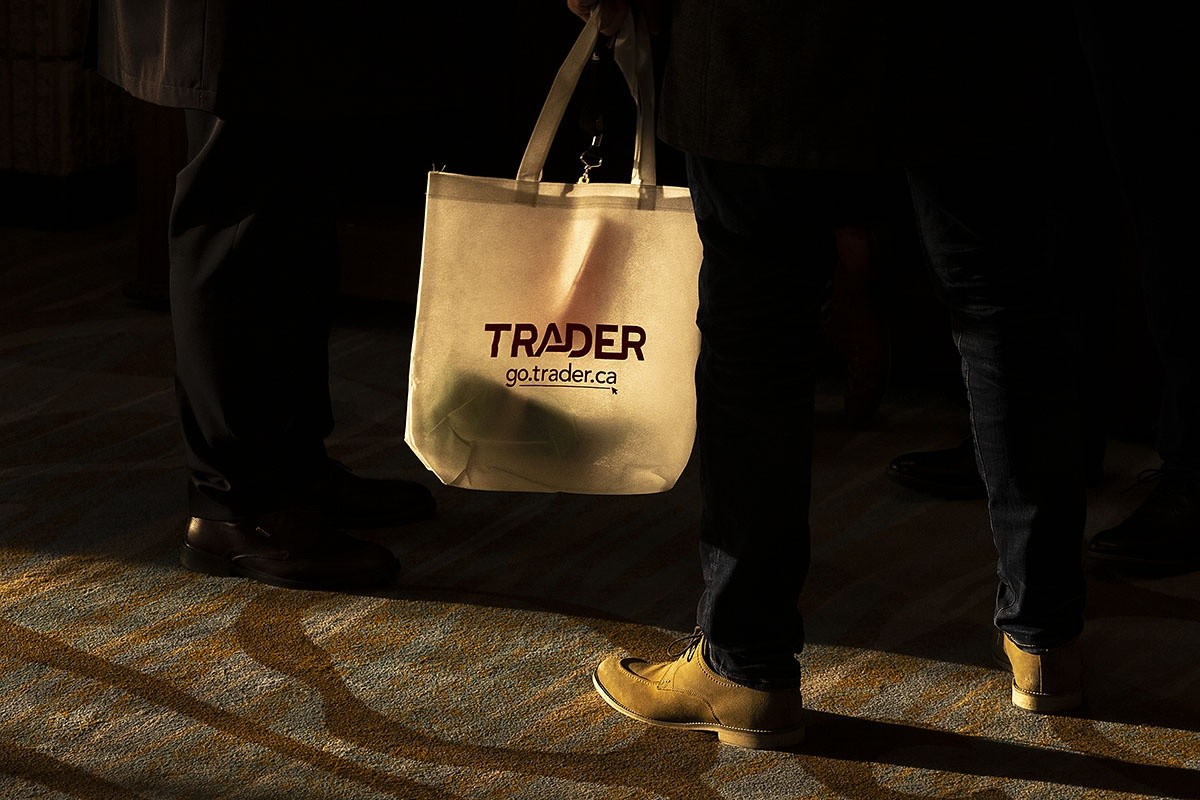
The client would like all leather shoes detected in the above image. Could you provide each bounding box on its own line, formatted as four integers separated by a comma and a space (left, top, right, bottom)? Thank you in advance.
993, 624, 1085, 712
885, 435, 989, 500
591, 626, 804, 749
179, 507, 401, 592
295, 462, 438, 531
1088, 466, 1200, 567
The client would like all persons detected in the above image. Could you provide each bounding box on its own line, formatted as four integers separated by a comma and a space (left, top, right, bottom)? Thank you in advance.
99, 0, 438, 594
566, 0, 1200, 747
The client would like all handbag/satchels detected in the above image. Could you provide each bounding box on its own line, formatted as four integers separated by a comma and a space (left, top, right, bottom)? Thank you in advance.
403, 0, 703, 495
